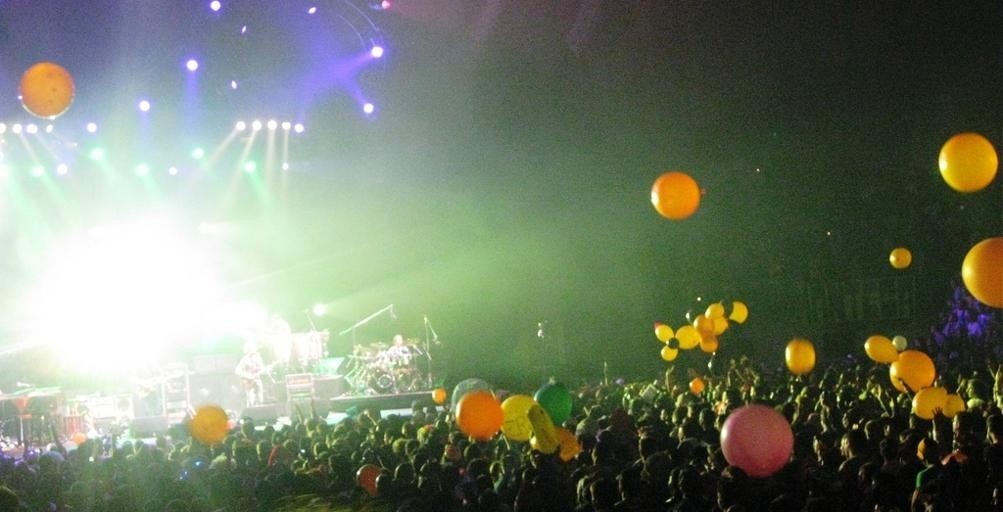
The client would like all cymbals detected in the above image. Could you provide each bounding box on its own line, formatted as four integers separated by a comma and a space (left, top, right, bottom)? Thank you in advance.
369, 343, 388, 350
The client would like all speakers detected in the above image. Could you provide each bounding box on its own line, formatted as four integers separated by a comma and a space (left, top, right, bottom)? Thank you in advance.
289, 398, 328, 420
128, 414, 168, 439
238, 404, 277, 426
346, 403, 381, 420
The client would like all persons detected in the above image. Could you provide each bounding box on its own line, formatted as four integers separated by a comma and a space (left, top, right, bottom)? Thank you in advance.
1, 333, 468, 511
916, 286, 1002, 510
463, 355, 917, 511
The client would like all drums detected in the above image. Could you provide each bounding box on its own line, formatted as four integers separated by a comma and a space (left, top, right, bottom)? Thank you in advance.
394, 368, 422, 393
371, 370, 393, 392
392, 356, 410, 370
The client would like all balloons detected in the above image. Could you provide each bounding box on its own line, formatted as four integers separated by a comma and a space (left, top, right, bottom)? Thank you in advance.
654, 298, 748, 361
18, 61, 76, 119
963, 236, 1002, 309
864, 335, 936, 391
889, 248, 912, 268
938, 131, 998, 194
649, 172, 701, 220
785, 338, 816, 373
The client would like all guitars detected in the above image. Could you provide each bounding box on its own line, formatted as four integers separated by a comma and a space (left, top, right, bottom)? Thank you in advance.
239, 361, 275, 392
